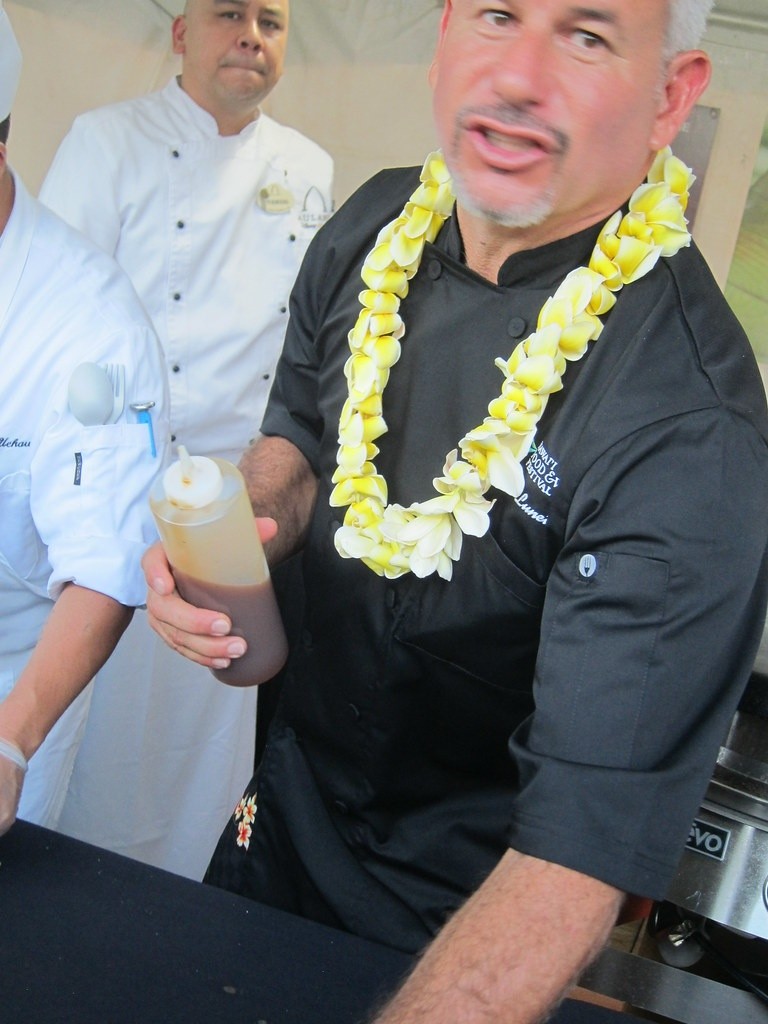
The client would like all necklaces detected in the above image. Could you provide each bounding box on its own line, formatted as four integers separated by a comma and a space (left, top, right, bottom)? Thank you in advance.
328, 145, 696, 583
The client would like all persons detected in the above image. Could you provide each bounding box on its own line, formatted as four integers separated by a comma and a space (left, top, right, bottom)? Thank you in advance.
38, 0, 336, 884
0, 0, 173, 836
142, 0, 768, 1024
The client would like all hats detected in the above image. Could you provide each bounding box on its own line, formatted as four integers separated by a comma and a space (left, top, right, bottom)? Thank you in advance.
0, 3, 22, 123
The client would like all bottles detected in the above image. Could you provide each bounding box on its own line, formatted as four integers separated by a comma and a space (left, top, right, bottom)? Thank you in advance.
150, 444, 289, 687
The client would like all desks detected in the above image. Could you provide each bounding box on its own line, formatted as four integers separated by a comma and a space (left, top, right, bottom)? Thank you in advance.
0, 820, 638, 1024
